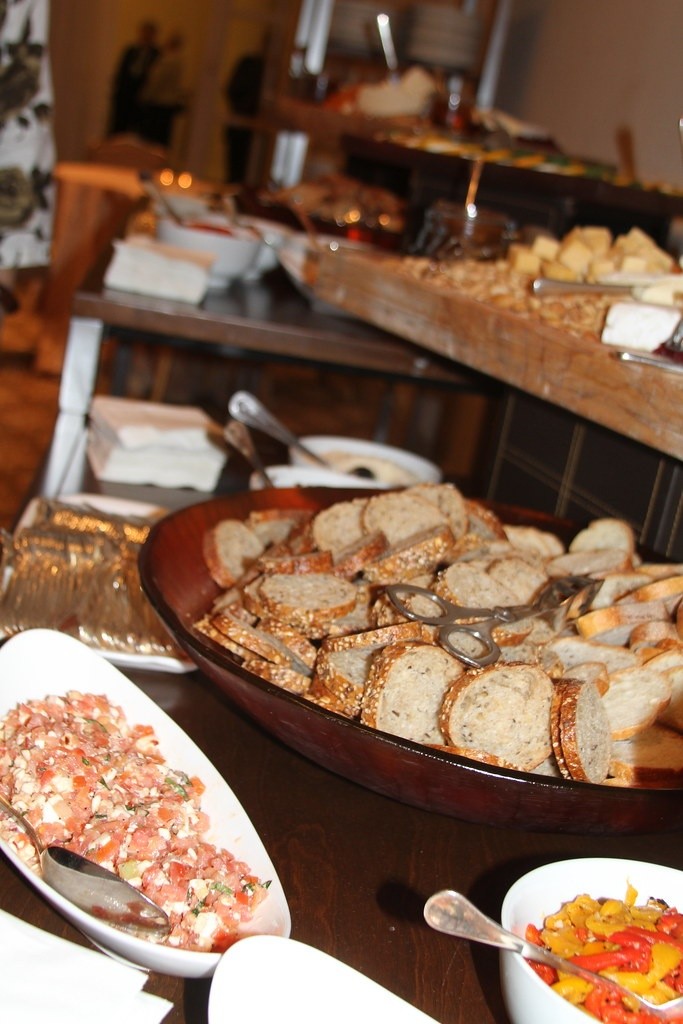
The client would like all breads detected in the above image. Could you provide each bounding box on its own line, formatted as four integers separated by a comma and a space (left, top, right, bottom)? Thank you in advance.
196, 481, 683, 792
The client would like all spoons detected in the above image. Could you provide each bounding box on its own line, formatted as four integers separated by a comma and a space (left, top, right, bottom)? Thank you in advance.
0, 498, 186, 659
423, 891, 683, 1018
227, 390, 376, 479
0, 796, 170, 934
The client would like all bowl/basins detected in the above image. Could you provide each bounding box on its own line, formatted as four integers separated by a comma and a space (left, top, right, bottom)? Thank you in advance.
159, 217, 261, 290
249, 464, 388, 489
501, 858, 683, 1024
279, 235, 367, 286
290, 434, 442, 485
231, 219, 289, 280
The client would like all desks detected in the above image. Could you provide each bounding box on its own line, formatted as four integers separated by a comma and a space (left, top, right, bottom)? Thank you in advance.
0, 230, 683, 1024
318, 133, 683, 290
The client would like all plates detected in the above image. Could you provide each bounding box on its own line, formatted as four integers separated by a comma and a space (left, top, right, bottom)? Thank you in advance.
137, 488, 683, 835
0, 629, 291, 978
209, 935, 441, 1024
0, 495, 198, 675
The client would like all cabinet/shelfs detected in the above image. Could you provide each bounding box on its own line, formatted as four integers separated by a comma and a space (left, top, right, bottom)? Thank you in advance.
35, 162, 237, 375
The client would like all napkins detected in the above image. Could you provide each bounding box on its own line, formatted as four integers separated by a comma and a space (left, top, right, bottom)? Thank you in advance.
86, 397, 229, 493
0, 908, 175, 1024
